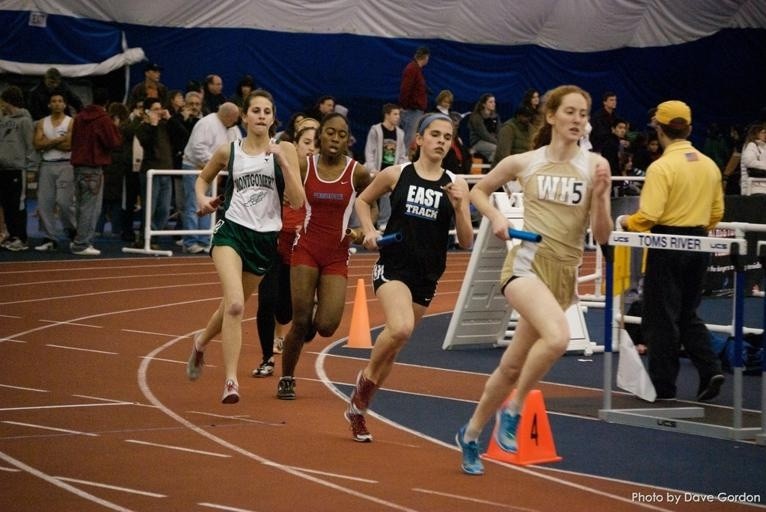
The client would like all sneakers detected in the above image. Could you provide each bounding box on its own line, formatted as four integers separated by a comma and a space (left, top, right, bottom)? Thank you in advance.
343, 408, 374, 443
492, 406, 523, 456
635, 369, 727, 403
186, 331, 206, 380
276, 375, 298, 402
251, 356, 275, 378
454, 423, 485, 476
220, 377, 241, 405
1, 238, 103, 256
351, 369, 379, 416
272, 335, 286, 354
182, 242, 203, 255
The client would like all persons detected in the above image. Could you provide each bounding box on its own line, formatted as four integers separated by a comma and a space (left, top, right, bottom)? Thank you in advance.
2, 46, 766, 476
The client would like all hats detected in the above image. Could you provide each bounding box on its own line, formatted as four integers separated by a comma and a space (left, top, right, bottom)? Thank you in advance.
653, 98, 694, 131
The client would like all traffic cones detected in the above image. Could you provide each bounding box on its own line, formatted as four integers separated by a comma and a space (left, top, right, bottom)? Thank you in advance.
342, 277, 373, 350
